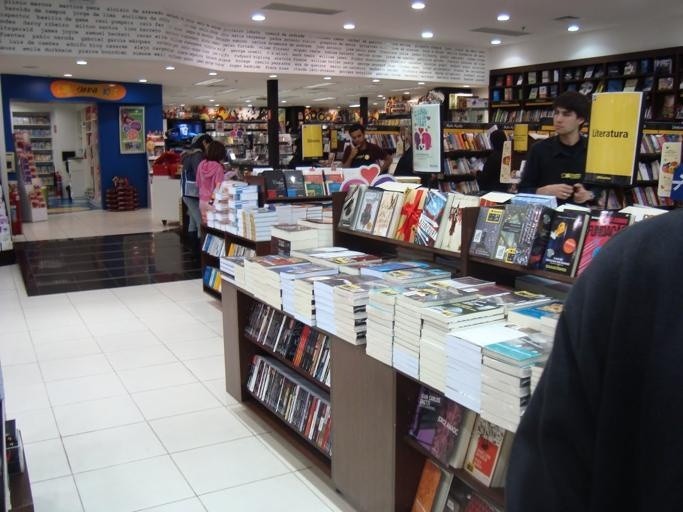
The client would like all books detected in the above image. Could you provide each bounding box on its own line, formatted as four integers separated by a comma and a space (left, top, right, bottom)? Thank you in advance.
220, 245, 566, 433
341, 177, 670, 304
441, 58, 682, 209
300, 382, 517, 511
0, 105, 101, 251
207, 121, 401, 165
202, 167, 344, 294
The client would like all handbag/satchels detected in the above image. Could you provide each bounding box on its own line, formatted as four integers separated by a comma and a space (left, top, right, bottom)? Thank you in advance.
184, 167, 199, 197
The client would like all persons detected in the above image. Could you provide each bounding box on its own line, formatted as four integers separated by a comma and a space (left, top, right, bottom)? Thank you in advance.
504, 203, 683, 511
179, 135, 231, 261
341, 122, 393, 174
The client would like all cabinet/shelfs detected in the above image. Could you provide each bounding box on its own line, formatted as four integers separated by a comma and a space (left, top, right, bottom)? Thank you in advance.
78, 106, 99, 204
1, 364, 34, 511
0, 111, 54, 266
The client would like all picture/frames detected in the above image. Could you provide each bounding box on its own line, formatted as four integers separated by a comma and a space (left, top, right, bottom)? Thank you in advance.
118, 107, 146, 155
